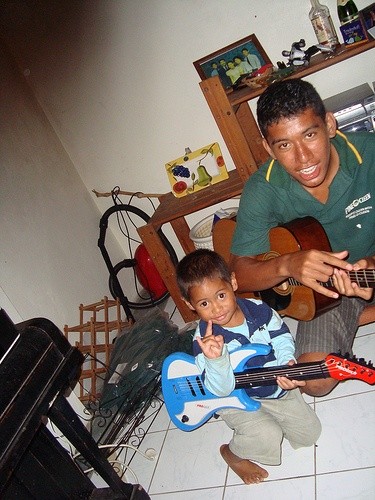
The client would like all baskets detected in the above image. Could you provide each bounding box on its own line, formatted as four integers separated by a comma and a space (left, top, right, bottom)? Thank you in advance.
245, 64, 273, 89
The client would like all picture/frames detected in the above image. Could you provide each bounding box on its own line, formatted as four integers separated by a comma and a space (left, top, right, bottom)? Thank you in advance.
192, 34, 272, 94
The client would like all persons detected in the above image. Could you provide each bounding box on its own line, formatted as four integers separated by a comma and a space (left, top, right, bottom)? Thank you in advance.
176, 248, 322, 484
282, 39, 336, 66
230, 78, 375, 396
211, 48, 261, 88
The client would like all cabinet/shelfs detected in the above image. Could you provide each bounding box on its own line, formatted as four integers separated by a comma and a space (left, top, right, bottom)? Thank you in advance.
64, 296, 127, 405
135, 39, 375, 325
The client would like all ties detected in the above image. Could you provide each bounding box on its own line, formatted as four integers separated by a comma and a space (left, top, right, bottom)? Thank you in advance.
247, 57, 252, 67
224, 65, 227, 69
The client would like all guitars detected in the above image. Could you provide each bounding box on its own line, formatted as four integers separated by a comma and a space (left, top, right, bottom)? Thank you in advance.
213, 217, 375, 321
160, 343, 375, 432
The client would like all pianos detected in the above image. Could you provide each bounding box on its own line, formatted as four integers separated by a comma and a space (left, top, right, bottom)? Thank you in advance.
0, 305, 151, 500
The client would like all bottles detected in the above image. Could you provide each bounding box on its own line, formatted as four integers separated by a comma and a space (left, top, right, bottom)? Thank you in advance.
308, 0, 342, 56
336, 0, 358, 23
